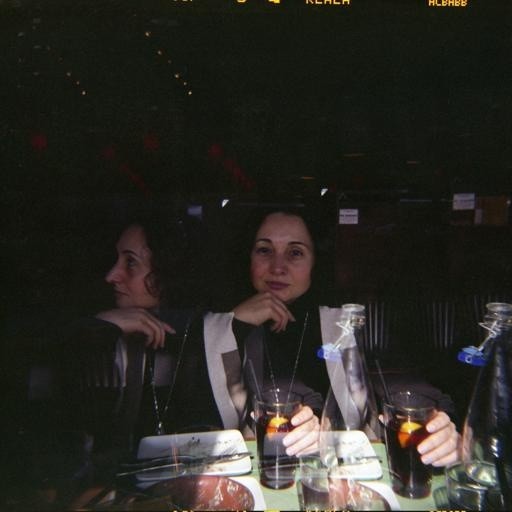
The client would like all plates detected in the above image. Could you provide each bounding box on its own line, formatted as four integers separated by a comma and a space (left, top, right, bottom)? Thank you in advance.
135, 429, 252, 481
264, 430, 383, 480
302, 479, 392, 512
131, 474, 255, 511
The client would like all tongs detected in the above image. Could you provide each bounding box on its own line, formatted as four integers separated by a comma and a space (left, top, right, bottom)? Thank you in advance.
116, 451, 252, 479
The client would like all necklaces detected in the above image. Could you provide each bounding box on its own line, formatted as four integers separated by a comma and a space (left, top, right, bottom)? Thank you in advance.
260, 310, 308, 418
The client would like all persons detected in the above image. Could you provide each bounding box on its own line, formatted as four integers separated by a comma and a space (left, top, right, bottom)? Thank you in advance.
53, 212, 221, 462
181, 204, 376, 437
373, 337, 461, 470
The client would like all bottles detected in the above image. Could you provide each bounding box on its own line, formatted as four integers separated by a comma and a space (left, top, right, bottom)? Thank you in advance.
461, 302, 511, 478
319, 305, 380, 478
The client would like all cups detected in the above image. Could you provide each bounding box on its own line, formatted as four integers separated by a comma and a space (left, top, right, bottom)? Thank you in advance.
384, 394, 434, 499
447, 460, 511, 512
254, 388, 303, 490
